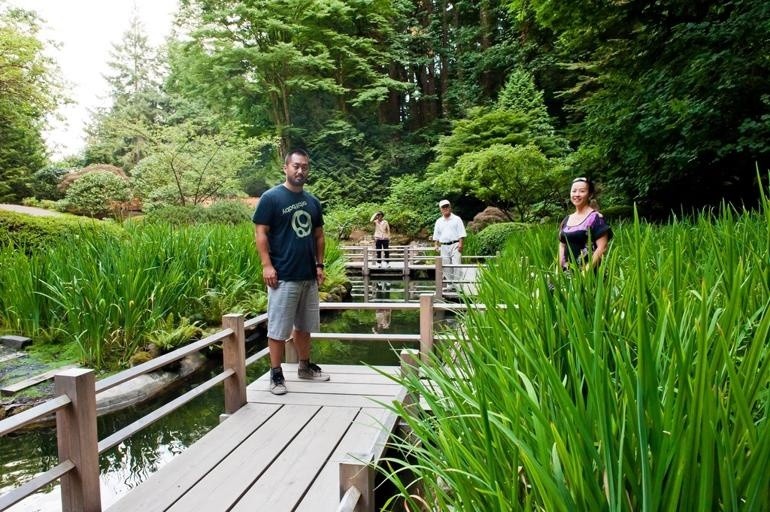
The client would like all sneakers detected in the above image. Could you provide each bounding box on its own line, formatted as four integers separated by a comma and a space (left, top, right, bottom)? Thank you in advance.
269, 368, 287, 395
298, 363, 330, 382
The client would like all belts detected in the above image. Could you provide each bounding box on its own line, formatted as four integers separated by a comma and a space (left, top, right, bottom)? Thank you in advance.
441, 240, 459, 246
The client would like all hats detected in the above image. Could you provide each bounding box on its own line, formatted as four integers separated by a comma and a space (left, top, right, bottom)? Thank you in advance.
439, 199, 451, 208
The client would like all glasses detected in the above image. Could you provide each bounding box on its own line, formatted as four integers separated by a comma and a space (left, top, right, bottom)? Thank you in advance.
572, 177, 590, 185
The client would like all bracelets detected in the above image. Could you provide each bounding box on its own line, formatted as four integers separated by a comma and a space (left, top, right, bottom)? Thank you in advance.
315, 263, 324, 268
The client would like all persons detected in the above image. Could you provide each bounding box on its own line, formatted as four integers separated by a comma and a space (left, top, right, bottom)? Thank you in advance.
370, 211, 391, 268
433, 199, 468, 291
254, 148, 331, 395
545, 177, 612, 293
372, 281, 393, 335
